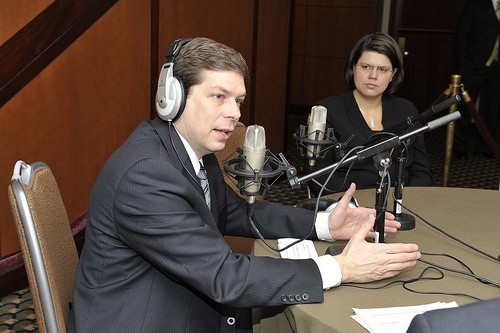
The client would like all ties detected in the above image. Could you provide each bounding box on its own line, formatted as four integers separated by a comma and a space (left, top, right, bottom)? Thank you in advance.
197, 161, 211, 211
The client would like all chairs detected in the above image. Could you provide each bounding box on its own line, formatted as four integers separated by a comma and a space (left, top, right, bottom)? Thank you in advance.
213, 121, 264, 203
7, 160, 80, 333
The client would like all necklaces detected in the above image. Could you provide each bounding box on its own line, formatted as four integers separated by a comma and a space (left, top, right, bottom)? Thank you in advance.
361, 110, 377, 128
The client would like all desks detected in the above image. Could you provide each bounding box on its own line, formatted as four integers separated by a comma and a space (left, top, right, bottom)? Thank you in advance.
253, 187, 500, 333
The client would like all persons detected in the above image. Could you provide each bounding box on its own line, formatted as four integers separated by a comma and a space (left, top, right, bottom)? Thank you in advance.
452, 0, 500, 160
65, 37, 421, 333
304, 33, 436, 196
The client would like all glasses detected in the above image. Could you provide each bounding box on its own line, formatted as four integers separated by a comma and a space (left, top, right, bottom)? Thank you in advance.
358, 63, 393, 73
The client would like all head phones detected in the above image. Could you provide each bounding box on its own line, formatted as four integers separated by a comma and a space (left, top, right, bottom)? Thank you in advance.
154, 36, 187, 123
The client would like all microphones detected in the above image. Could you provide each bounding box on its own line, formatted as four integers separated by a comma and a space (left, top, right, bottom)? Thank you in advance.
240, 123, 267, 217
306, 105, 328, 173
